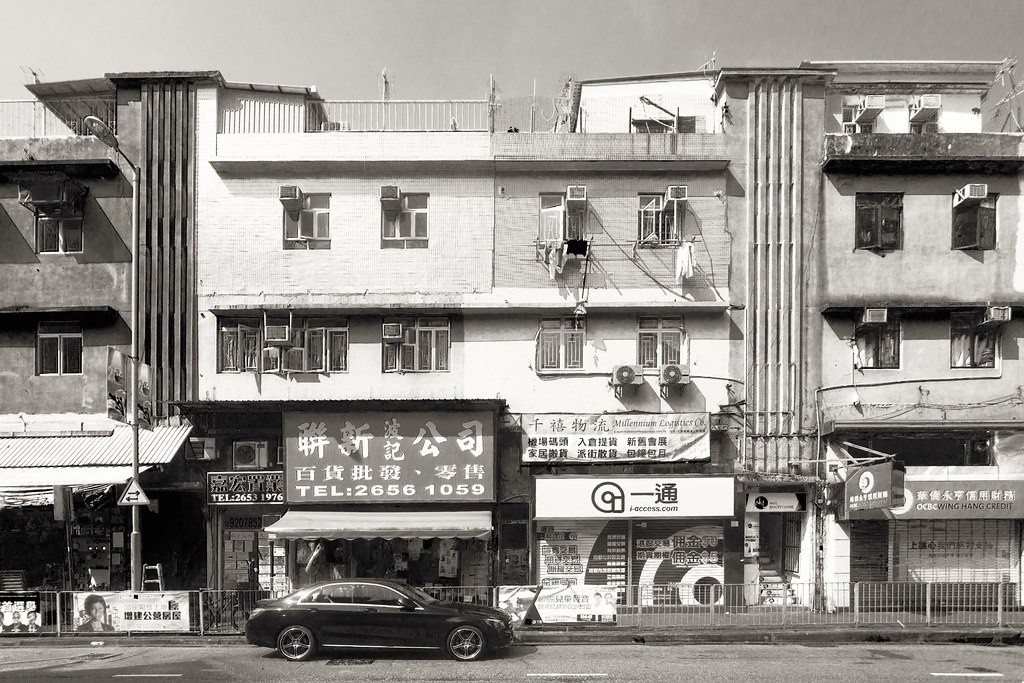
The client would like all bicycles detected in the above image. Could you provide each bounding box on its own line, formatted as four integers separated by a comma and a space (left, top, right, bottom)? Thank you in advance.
190, 586, 257, 632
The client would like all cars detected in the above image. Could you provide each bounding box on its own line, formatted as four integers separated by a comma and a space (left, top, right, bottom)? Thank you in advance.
244, 578, 514, 662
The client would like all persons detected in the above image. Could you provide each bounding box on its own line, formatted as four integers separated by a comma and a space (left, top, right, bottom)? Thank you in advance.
76, 594, 115, 632
0, 611, 42, 634
603, 593, 616, 614
594, 593, 603, 615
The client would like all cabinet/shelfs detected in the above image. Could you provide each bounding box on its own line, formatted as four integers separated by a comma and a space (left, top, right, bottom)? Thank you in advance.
70, 535, 112, 591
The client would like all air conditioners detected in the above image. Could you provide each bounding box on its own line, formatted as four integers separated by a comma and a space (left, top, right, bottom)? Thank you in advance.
185, 437, 216, 460
659, 365, 690, 385
612, 364, 643, 384
233, 441, 268, 470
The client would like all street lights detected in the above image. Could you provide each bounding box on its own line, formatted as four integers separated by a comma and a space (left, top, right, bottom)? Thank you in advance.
83, 116, 150, 591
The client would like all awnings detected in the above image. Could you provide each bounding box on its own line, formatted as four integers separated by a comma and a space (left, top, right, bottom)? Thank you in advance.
158, 397, 509, 432
263, 511, 494, 541
1, 424, 192, 508
821, 417, 1024, 438
